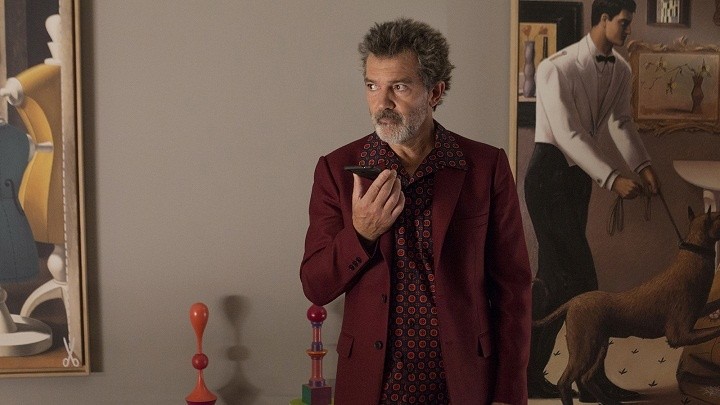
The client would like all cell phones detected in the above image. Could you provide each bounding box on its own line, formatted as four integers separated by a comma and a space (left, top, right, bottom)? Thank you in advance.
343, 166, 383, 181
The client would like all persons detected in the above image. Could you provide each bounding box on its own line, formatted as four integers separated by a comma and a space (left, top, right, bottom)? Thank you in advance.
299, 19, 532, 405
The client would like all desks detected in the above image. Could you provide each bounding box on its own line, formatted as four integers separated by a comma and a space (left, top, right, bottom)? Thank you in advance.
672, 159, 720, 270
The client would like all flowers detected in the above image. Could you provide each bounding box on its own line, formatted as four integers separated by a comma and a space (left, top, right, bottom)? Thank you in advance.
522, 23, 547, 40
644, 56, 711, 94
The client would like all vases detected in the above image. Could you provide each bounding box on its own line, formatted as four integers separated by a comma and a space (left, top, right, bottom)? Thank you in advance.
692, 76, 705, 115
523, 41, 536, 97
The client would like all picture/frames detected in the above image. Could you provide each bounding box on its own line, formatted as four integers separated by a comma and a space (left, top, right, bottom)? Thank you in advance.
518, 0, 582, 128
625, 35, 720, 134
647, 0, 689, 27
0, 0, 92, 378
